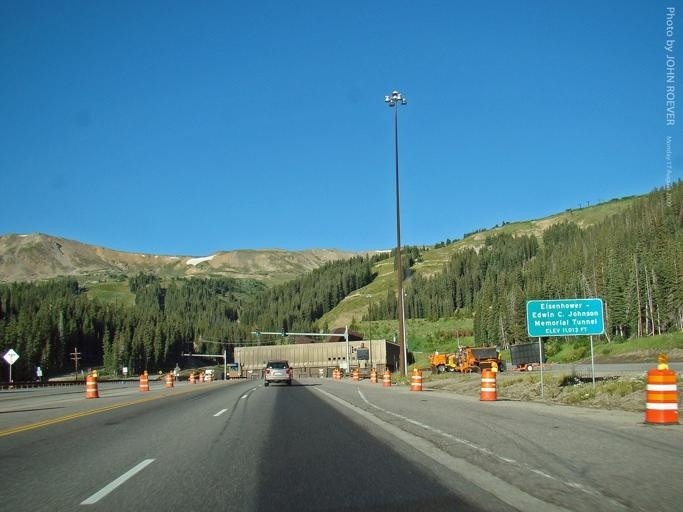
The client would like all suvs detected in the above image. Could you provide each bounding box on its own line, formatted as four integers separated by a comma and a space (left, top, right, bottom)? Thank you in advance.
262, 360, 292, 387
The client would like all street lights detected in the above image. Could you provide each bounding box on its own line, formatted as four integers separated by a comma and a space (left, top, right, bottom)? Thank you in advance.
447, 329, 459, 349
384, 90, 407, 380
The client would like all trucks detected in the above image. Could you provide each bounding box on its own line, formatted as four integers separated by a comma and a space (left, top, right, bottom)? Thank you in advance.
203, 369, 224, 382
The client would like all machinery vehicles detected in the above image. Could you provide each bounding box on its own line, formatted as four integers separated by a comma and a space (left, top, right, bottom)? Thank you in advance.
431, 353, 461, 373
454, 347, 504, 374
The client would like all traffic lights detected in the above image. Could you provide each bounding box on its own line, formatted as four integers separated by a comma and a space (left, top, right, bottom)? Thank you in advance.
256, 328, 260, 337
281, 326, 286, 337
